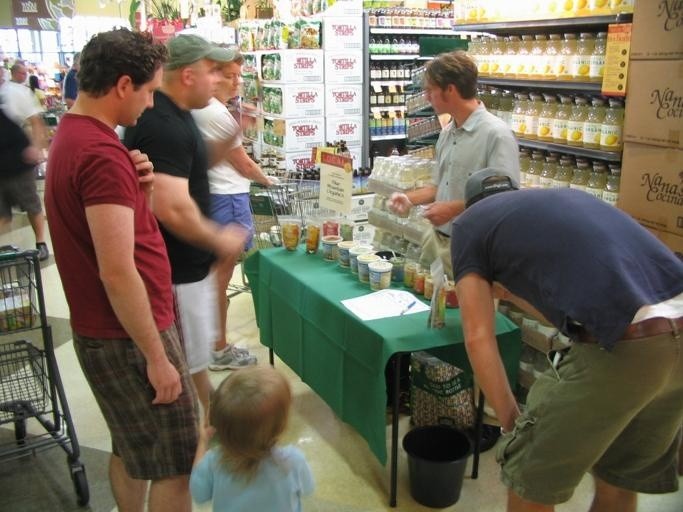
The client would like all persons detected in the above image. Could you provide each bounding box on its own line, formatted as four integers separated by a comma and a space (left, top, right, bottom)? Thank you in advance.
0, 50, 88, 262
451, 167, 683, 512
188, 43, 281, 374
382, 51, 520, 286
188, 363, 314, 511
44, 29, 205, 512
121, 35, 251, 427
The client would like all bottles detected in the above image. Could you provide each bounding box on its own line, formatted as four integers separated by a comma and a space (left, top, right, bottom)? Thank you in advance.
370, 6, 455, 29
371, 152, 438, 190
477, 87, 623, 153
515, 144, 623, 209
369, 196, 434, 260
368, 85, 405, 107
466, 29, 607, 81
367, 59, 418, 82
268, 224, 281, 249
388, 255, 458, 308
406, 65, 443, 157
367, 34, 420, 55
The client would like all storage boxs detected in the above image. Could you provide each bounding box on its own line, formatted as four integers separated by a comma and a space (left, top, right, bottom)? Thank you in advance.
615, 0, 682, 254
225, 14, 361, 180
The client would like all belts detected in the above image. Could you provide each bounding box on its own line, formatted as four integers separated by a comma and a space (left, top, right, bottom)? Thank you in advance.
625, 316, 682, 340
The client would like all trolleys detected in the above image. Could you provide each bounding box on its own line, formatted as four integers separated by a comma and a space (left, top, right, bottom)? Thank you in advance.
28, 115, 59, 192
0, 243, 91, 509
224, 176, 320, 304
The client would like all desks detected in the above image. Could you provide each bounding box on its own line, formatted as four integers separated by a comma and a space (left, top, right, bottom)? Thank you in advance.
246, 244, 521, 507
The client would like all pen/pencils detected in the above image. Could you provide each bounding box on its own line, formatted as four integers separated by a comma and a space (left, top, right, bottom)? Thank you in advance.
401, 301, 416, 314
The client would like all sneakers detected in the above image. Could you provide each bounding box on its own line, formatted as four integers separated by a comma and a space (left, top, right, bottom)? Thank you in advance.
207, 347, 257, 371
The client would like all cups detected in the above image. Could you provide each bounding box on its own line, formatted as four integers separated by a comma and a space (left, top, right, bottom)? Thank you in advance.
281, 224, 300, 251
304, 226, 319, 254
319, 234, 396, 290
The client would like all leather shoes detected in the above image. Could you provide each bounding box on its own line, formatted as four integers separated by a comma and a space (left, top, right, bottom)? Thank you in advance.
36, 242, 48, 262
471, 424, 499, 453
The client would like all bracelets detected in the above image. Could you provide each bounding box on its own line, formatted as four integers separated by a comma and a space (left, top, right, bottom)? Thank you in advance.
501, 425, 512, 436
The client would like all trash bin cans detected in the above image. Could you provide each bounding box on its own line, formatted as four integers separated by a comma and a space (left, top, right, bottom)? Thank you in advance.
402, 416, 471, 509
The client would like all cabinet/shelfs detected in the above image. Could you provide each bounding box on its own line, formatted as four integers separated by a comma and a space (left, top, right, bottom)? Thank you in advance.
450, 11, 633, 169
362, 5, 453, 172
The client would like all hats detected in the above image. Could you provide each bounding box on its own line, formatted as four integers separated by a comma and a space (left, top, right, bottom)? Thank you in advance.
464, 167, 519, 206
163, 34, 235, 69
